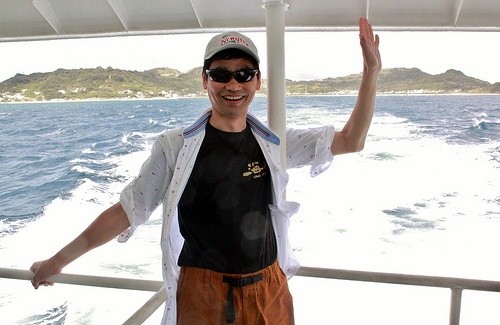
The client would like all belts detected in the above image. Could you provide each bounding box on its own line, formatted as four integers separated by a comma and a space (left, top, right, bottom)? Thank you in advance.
222, 274, 262, 323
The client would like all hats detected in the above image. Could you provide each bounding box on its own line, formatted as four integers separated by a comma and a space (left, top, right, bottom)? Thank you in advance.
204, 32, 260, 63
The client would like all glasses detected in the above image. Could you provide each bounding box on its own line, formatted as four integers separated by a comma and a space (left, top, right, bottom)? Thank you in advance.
205, 68, 259, 84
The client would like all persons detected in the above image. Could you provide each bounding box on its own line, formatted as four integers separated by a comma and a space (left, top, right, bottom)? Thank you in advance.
30, 16, 382, 325
